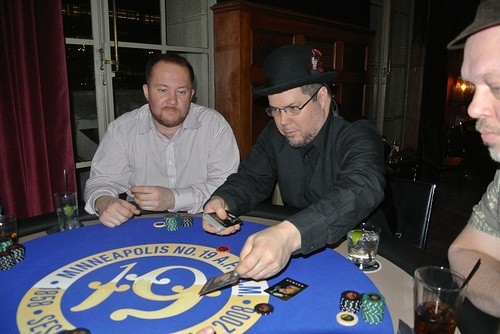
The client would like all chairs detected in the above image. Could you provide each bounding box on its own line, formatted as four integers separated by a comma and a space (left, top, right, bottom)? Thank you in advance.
387, 177, 437, 251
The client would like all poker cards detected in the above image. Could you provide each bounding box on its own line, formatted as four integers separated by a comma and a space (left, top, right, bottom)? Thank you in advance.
264, 277, 310, 302
202, 209, 243, 231
199, 270, 240, 296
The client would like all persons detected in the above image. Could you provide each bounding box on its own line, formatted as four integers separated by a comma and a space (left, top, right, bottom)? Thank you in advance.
201, 45, 398, 281
84, 54, 240, 227
446, 1, 500, 320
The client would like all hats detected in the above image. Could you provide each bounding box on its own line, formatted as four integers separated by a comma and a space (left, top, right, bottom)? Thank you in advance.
251, 44, 337, 96
447, 0, 500, 50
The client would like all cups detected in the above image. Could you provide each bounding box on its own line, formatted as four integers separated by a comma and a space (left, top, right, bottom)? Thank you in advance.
54, 191, 79, 231
346, 223, 381, 266
413, 266, 468, 334
0, 214, 19, 256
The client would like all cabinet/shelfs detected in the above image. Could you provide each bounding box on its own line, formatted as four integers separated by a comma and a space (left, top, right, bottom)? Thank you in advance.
210, 0, 377, 163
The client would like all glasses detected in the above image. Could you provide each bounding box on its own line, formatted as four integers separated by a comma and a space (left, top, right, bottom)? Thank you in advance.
265, 84, 329, 117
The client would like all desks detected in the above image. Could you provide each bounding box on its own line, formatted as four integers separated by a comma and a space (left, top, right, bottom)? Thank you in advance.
0, 205, 500, 334
